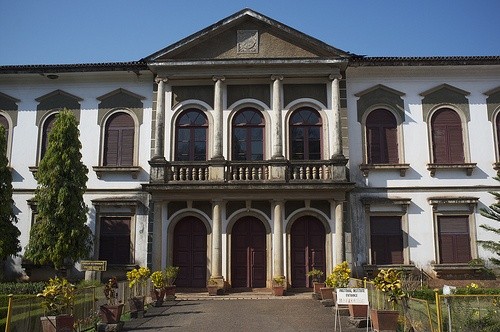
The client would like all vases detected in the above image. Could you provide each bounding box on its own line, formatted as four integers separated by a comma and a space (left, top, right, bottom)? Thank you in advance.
320, 277, 333, 300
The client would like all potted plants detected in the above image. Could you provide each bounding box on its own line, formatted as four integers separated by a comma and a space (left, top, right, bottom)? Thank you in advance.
151, 271, 164, 306
306, 268, 325, 294
274, 275, 286, 296
348, 279, 368, 317
207, 279, 218, 295
164, 266, 179, 298
36, 278, 74, 332
331, 261, 351, 305
125, 266, 151, 316
100, 276, 124, 323
370, 267, 406, 332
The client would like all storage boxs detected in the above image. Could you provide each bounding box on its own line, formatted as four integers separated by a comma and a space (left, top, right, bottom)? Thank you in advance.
336, 288, 369, 305
